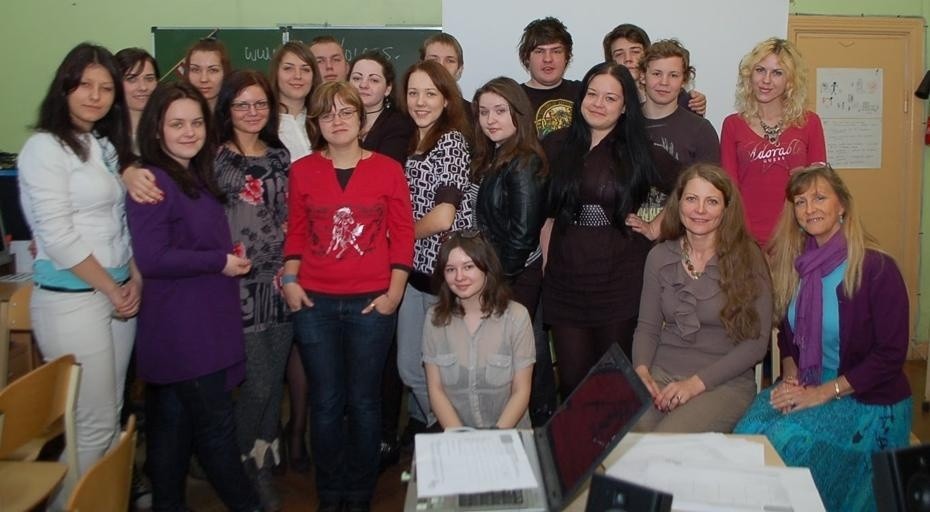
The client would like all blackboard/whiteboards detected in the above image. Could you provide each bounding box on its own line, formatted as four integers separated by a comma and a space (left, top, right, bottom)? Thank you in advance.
150, 25, 443, 99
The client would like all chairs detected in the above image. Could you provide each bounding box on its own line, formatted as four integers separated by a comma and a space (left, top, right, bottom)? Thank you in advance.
0, 353, 84, 512
65, 413, 137, 512
0, 281, 34, 390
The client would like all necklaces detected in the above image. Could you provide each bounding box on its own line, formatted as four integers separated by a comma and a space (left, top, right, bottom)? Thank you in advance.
365, 106, 384, 114
755, 112, 785, 144
682, 236, 707, 280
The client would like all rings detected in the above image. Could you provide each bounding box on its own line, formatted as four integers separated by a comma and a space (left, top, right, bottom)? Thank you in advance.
787, 388, 789, 393
369, 303, 376, 310
676, 395, 680, 401
641, 221, 643, 225
788, 399, 791, 406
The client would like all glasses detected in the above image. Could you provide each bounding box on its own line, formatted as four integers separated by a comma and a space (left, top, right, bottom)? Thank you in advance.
230, 101, 269, 110
319, 107, 357, 121
437, 229, 483, 242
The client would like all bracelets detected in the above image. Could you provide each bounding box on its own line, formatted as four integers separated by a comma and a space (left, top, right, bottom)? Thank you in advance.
281, 274, 297, 283
833, 378, 842, 399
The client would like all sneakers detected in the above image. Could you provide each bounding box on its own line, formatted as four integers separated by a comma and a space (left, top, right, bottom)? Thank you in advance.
132, 475, 153, 511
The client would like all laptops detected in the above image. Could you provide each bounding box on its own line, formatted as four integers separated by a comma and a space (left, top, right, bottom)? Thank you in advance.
403, 342, 655, 512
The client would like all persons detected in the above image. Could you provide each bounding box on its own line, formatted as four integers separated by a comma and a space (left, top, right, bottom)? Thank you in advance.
419, 32, 473, 121
124, 79, 259, 512
471, 76, 551, 287
16, 42, 143, 511
346, 50, 415, 169
518, 17, 587, 143
308, 34, 351, 85
269, 40, 323, 473
734, 169, 914, 512
398, 59, 472, 447
185, 39, 232, 142
719, 38, 828, 254
603, 25, 708, 118
632, 162, 776, 433
544, 60, 684, 406
421, 224, 538, 430
209, 70, 298, 511
111, 46, 160, 173
281, 79, 416, 511
634, 40, 722, 170
